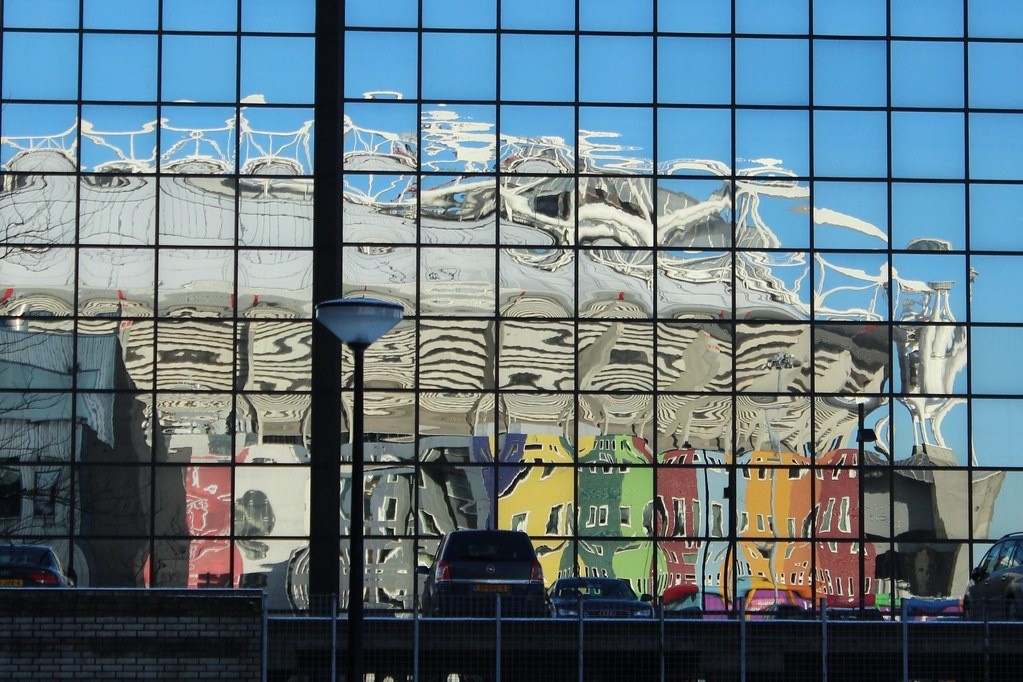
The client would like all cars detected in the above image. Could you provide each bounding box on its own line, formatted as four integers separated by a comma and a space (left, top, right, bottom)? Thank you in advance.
820, 605, 883, 620
0, 544, 75, 590
545, 577, 653, 621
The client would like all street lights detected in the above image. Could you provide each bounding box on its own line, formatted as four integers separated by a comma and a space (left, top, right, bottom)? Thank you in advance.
305, 296, 407, 682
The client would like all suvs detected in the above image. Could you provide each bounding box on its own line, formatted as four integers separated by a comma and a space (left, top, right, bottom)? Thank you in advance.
414, 528, 547, 618
962, 531, 1023, 622
